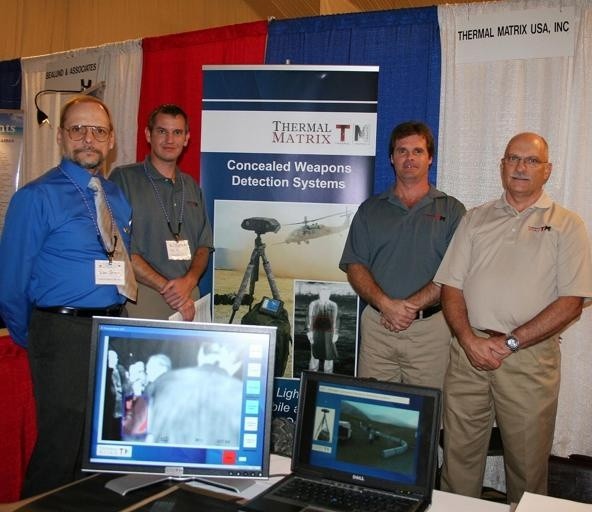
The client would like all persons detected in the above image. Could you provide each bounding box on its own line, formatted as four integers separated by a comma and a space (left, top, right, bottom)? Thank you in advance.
109, 105, 216, 321
432, 133, 592, 504
340, 122, 467, 430
306, 289, 340, 374
1, 95, 132, 501
102, 338, 243, 447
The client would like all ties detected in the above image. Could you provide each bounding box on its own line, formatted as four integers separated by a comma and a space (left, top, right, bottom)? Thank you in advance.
88, 176, 138, 303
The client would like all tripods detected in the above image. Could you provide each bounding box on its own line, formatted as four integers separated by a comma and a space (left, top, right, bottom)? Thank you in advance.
229, 244, 285, 324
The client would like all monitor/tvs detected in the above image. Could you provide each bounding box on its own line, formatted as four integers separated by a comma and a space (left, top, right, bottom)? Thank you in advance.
79, 314, 278, 493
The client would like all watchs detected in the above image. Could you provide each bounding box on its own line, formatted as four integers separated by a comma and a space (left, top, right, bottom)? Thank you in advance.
505, 332, 519, 353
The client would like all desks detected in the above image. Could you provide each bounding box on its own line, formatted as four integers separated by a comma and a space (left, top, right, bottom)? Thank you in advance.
0, 453, 592, 512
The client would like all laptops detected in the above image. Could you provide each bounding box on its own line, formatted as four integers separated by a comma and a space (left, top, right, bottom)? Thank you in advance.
242, 367, 443, 512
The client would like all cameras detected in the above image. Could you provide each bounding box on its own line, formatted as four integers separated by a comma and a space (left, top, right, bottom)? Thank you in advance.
241, 211, 281, 237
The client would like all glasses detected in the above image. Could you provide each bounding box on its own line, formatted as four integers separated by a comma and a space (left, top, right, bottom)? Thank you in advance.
63, 124, 111, 143
502, 155, 549, 168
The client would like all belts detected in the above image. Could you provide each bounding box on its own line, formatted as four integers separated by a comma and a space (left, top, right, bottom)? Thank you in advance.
32, 304, 123, 317
473, 326, 506, 337
370, 304, 443, 320
